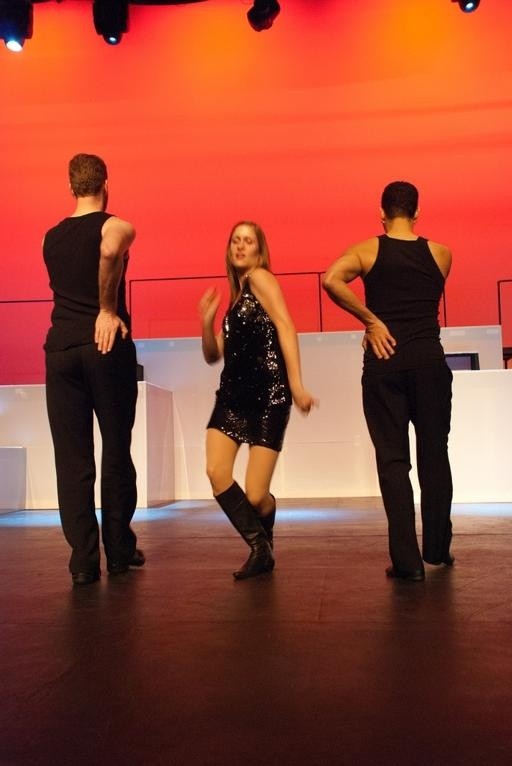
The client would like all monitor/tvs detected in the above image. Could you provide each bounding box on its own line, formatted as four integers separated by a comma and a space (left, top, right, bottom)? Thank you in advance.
442, 352, 480, 372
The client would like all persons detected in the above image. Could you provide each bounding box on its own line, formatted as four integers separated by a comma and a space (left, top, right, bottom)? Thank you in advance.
322, 182, 454, 582
200, 221, 320, 580
42, 154, 146, 586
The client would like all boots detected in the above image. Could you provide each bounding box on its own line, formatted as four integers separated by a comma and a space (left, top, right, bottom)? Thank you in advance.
214, 481, 274, 578
256, 493, 276, 549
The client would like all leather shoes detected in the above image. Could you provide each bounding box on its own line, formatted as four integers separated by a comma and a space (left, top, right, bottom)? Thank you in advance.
73, 549, 145, 584
386, 552, 454, 580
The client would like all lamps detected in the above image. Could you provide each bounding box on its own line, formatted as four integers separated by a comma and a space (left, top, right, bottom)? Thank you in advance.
244, 1, 282, 36
90, 1, 129, 46
0, 2, 35, 55
